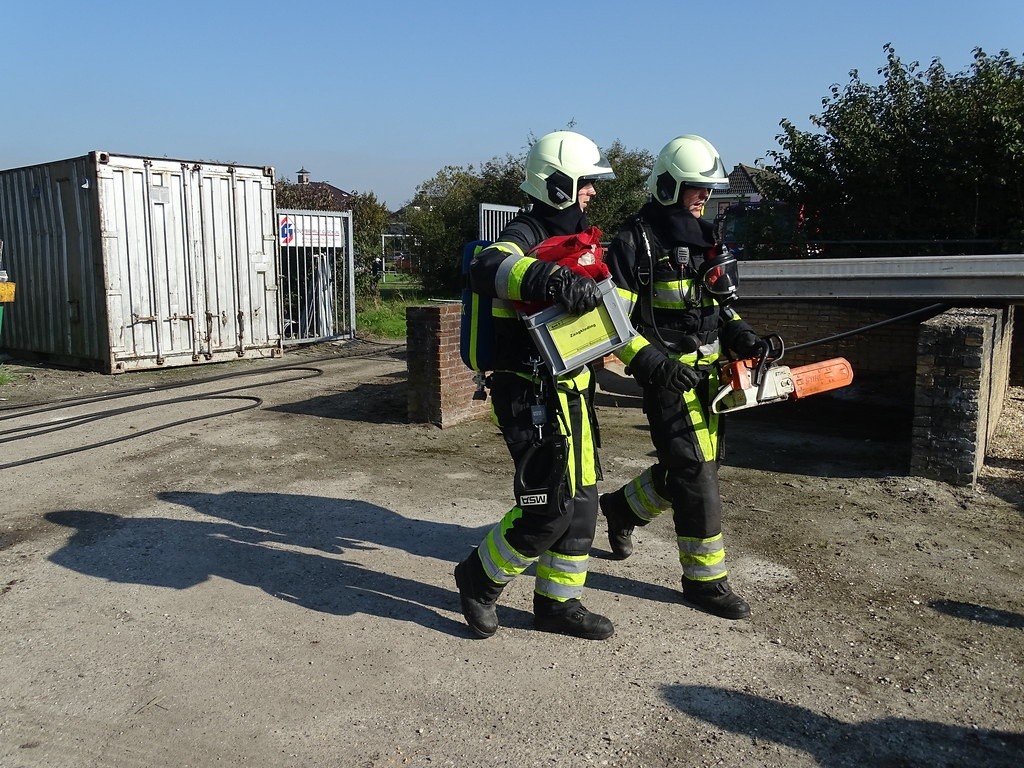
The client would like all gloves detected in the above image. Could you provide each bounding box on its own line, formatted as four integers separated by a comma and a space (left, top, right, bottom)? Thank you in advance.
624, 345, 698, 398
720, 321, 764, 359
544, 265, 604, 316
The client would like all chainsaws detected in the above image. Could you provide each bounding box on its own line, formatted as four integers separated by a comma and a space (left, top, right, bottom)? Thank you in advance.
711, 331, 855, 415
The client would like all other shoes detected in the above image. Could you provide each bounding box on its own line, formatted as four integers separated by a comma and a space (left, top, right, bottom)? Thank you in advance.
456, 551, 505, 637
533, 594, 614, 638
600, 489, 649, 558
682, 577, 750, 617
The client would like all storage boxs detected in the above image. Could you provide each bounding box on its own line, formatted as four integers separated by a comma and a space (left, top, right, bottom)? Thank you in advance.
516, 271, 637, 378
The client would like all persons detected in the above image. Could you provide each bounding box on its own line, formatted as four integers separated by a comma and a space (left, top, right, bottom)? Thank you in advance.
598, 134, 770, 620
454, 131, 615, 640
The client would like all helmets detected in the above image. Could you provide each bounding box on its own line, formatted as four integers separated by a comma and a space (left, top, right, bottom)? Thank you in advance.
648, 135, 727, 205
519, 132, 616, 208
